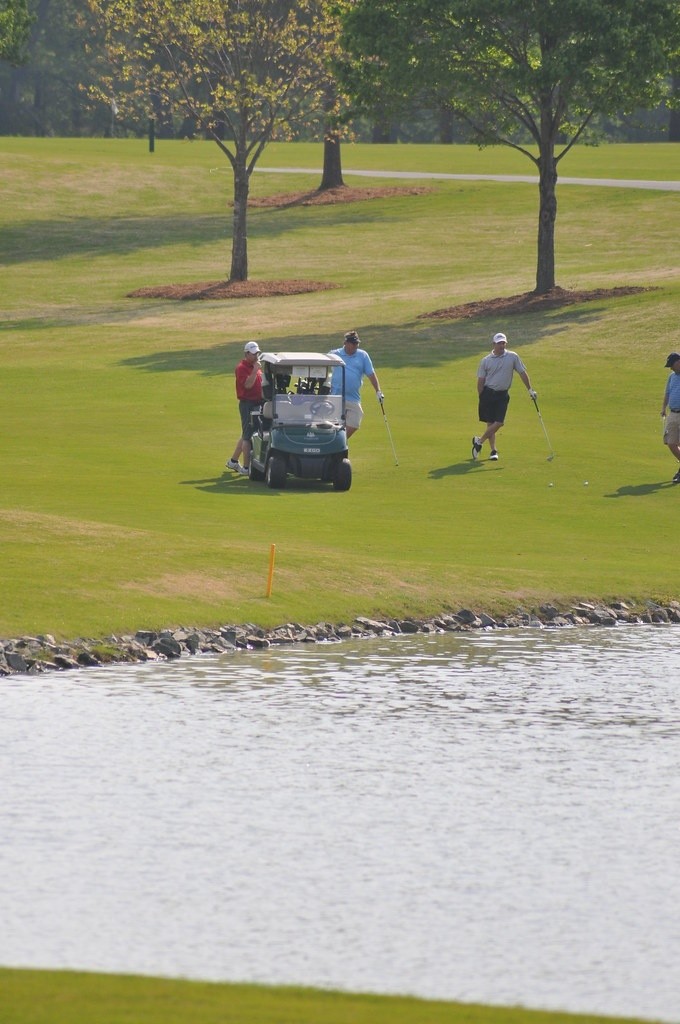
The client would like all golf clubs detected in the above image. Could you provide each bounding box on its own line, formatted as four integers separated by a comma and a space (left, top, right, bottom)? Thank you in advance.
380, 398, 400, 466
532, 395, 554, 461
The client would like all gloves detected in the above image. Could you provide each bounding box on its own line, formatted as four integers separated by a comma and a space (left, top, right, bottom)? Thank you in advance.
528, 389, 537, 399
376, 391, 384, 404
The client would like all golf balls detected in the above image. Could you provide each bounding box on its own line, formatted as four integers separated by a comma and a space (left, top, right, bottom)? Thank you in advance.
549, 483, 553, 487
584, 481, 589, 486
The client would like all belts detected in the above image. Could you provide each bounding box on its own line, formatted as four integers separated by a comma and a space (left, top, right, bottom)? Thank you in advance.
671, 410, 680, 413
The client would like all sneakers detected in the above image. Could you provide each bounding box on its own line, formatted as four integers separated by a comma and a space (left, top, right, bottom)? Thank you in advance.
472, 437, 483, 460
225, 460, 242, 472
240, 467, 249, 476
673, 468, 680, 481
489, 449, 498, 460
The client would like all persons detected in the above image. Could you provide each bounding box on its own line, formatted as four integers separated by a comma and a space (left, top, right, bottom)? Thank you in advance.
326, 331, 384, 439
225, 342, 263, 476
660, 353, 680, 483
472, 333, 538, 461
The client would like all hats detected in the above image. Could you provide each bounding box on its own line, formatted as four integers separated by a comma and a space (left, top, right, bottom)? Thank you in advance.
244, 342, 261, 354
665, 353, 680, 367
344, 331, 361, 344
493, 333, 507, 344
